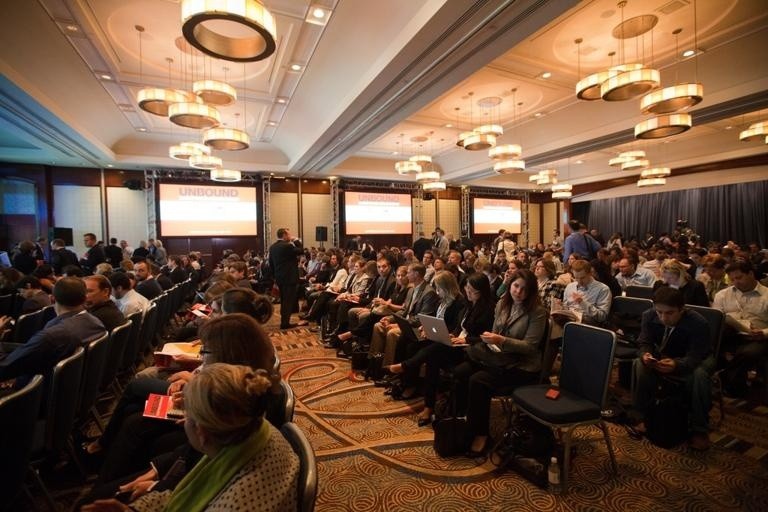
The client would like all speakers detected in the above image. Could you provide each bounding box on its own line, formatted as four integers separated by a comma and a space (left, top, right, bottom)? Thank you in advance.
49, 227, 72, 246
316, 227, 327, 241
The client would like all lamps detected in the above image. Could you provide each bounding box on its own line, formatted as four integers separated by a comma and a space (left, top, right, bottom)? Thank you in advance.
135, 0, 277, 185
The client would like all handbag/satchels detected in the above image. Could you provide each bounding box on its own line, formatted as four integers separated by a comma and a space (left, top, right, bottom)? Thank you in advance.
432, 417, 473, 457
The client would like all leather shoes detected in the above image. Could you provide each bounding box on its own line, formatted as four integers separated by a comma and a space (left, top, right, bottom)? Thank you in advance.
464, 435, 495, 458
375, 377, 399, 386
281, 322, 297, 329
380, 363, 405, 375
625, 420, 649, 440
300, 314, 379, 373
399, 385, 420, 400
419, 409, 433, 425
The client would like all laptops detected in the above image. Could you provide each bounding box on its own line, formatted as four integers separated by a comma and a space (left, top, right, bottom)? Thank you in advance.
417, 314, 471, 347
393, 313, 429, 344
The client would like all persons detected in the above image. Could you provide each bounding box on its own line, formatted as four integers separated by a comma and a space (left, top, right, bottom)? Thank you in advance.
78, 312, 288, 510
78, 362, 302, 510
0, 221, 768, 476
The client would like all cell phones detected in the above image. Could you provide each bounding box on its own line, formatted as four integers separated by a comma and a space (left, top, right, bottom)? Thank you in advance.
647, 357, 659, 366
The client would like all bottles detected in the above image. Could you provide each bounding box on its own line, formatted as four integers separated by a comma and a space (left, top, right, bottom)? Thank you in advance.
547, 456, 561, 496
